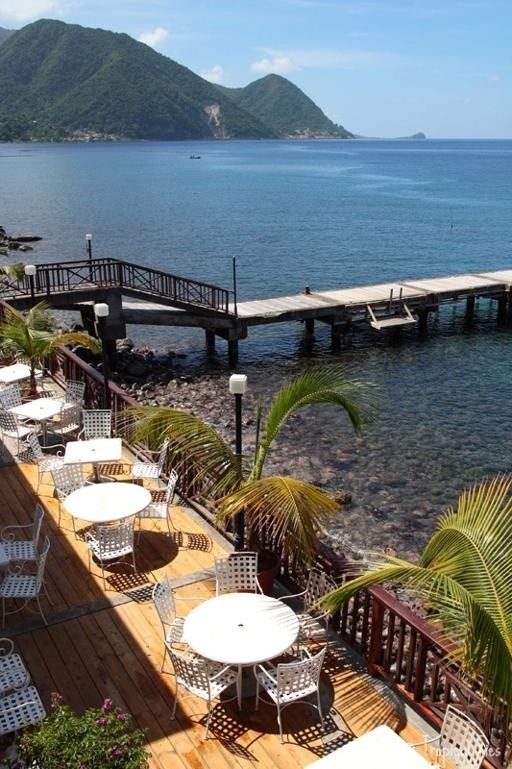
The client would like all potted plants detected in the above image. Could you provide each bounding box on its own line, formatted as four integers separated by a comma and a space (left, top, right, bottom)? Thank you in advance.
110, 362, 384, 595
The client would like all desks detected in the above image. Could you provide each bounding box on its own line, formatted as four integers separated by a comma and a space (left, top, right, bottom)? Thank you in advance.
301, 724, 435, 769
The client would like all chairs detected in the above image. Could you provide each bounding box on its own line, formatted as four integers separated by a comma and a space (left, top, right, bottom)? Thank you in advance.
410, 703, 491, 769
151, 550, 339, 744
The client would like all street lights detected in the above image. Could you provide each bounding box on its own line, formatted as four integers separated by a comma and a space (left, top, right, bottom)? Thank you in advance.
93, 302, 113, 412
228, 373, 249, 552
85, 233, 94, 282
25, 264, 38, 309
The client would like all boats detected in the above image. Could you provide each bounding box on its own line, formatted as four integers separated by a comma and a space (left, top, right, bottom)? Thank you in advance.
190, 154, 201, 159
